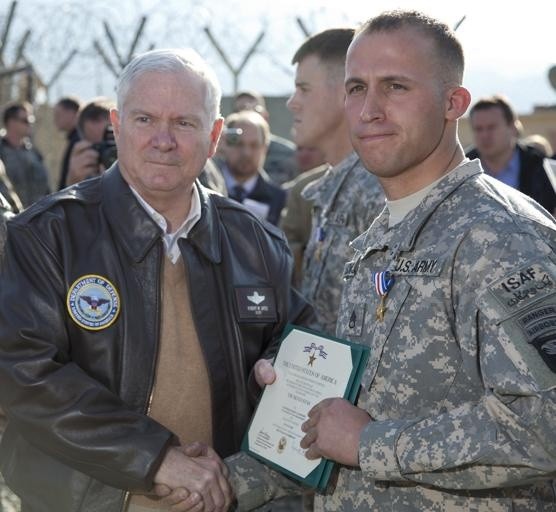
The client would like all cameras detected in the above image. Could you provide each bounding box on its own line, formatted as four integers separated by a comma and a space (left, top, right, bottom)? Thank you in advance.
94, 124, 117, 167
222, 128, 243, 146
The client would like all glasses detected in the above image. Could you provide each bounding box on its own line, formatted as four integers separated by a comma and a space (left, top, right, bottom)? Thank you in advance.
14, 116, 36, 124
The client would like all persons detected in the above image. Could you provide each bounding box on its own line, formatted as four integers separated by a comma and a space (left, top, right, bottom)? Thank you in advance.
64, 97, 116, 186
464, 93, 554, 212
223, 112, 286, 225
148, 8, 556, 512
1, 162, 24, 512
198, 159, 228, 198
2, 46, 325, 512
231, 92, 297, 184
54, 97, 81, 191
2, 100, 51, 214
277, 24, 360, 300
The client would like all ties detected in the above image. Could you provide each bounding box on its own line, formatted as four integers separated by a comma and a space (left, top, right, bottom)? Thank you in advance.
233, 186, 245, 203
